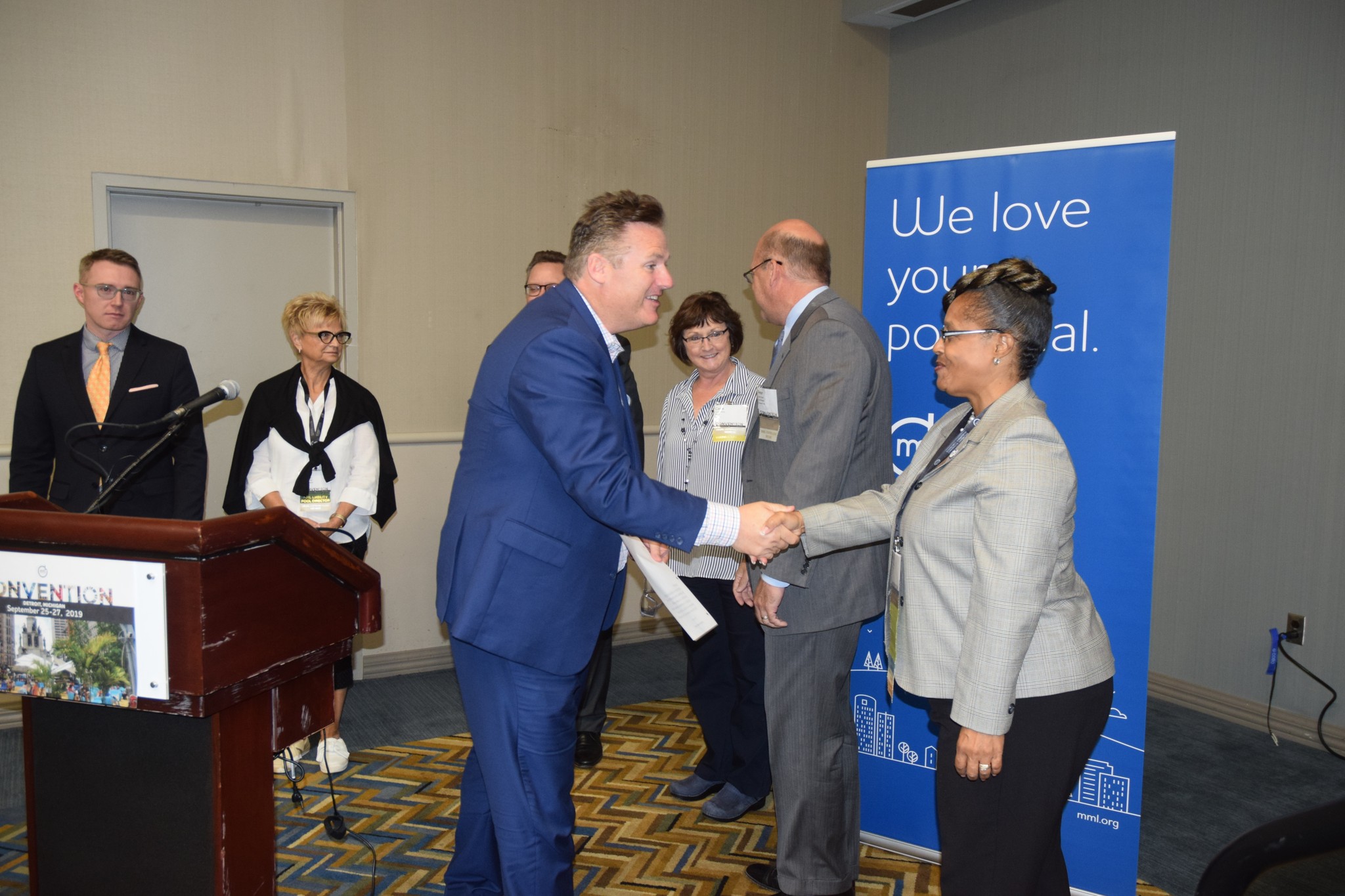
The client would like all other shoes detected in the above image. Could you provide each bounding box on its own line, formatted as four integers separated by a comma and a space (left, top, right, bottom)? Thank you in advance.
746, 861, 856, 896
668, 772, 725, 801
702, 781, 766, 823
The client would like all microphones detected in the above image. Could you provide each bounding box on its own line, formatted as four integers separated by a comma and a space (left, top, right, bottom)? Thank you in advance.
162, 379, 241, 422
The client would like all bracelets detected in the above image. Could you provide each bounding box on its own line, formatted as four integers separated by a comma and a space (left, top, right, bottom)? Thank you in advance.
329, 513, 348, 529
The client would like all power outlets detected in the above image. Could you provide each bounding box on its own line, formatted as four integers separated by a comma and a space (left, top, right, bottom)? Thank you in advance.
1285, 612, 1306, 646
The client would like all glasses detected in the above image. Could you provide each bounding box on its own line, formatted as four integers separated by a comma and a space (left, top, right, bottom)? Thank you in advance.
682, 327, 728, 344
941, 328, 1004, 342
524, 283, 559, 297
640, 547, 669, 618
303, 330, 352, 344
80, 281, 144, 303
743, 258, 782, 283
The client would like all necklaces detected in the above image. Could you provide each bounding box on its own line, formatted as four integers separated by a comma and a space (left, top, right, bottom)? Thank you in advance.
680, 401, 718, 494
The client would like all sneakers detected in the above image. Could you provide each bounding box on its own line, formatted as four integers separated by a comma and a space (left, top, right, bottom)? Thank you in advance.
318, 737, 350, 773
274, 734, 311, 774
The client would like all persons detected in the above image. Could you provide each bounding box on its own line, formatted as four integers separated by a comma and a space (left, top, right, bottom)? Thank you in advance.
432, 187, 800, 896
745, 258, 1113, 896
522, 249, 644, 769
0, 671, 129, 706
647, 291, 771, 823
9, 249, 209, 526
733, 218, 894, 896
222, 294, 397, 775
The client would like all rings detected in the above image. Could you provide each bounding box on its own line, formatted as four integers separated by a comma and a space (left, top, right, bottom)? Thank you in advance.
979, 763, 991, 770
761, 616, 768, 620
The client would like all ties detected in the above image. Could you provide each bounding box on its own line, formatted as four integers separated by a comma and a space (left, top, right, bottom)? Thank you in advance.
85, 342, 120, 430
770, 327, 785, 371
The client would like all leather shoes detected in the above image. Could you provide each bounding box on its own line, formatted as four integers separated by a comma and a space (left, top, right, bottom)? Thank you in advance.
573, 730, 604, 767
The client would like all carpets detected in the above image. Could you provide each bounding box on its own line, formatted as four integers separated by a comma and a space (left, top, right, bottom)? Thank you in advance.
1, 697, 1165, 896
